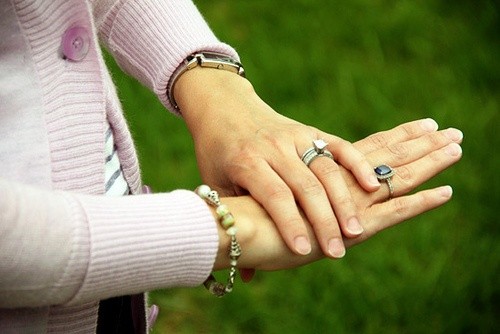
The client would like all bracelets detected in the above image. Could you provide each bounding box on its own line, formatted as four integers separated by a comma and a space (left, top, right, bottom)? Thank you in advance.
191, 184, 240, 300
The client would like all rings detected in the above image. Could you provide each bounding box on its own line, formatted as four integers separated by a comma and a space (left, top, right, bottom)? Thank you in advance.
372, 165, 394, 198
301, 138, 334, 167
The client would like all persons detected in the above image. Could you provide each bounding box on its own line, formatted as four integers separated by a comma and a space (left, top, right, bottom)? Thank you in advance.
0, 0, 464, 334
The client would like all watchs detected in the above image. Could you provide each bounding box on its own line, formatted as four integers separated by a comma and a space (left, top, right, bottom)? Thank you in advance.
166, 52, 247, 110
